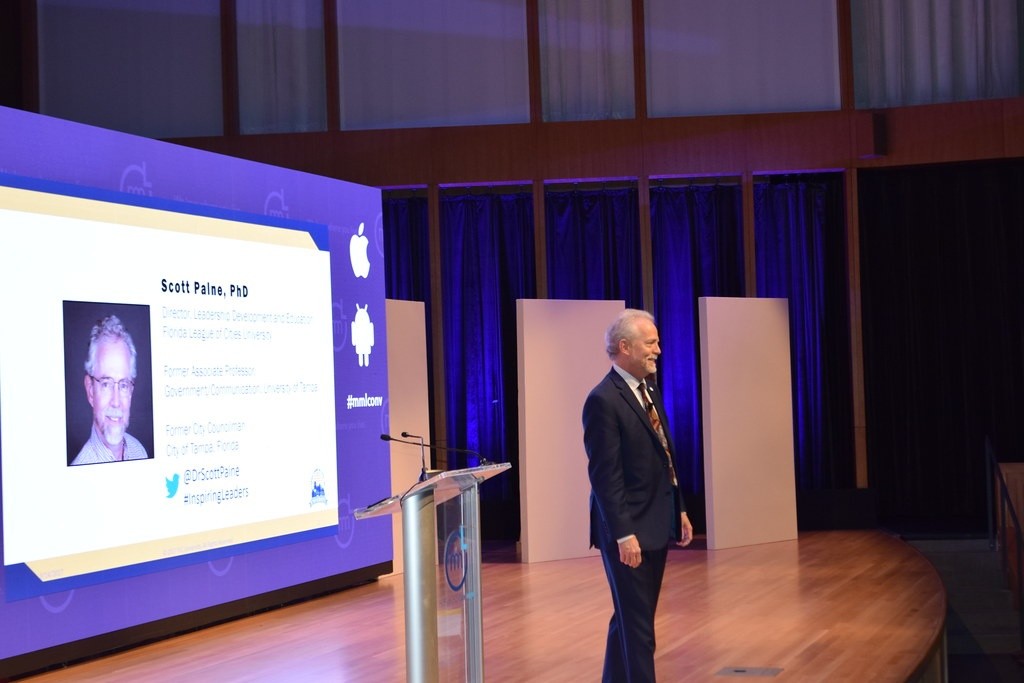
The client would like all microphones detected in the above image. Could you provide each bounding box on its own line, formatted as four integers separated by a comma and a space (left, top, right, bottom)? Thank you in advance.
401, 432, 425, 473
379, 433, 487, 464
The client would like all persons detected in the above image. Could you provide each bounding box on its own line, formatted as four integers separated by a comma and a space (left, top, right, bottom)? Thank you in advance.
580, 307, 695, 683
70, 315, 149, 465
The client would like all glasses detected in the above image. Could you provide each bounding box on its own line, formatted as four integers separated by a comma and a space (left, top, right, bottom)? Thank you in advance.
89, 375, 135, 397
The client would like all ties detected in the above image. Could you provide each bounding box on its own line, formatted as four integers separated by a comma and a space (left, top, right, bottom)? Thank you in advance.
636, 383, 678, 486
